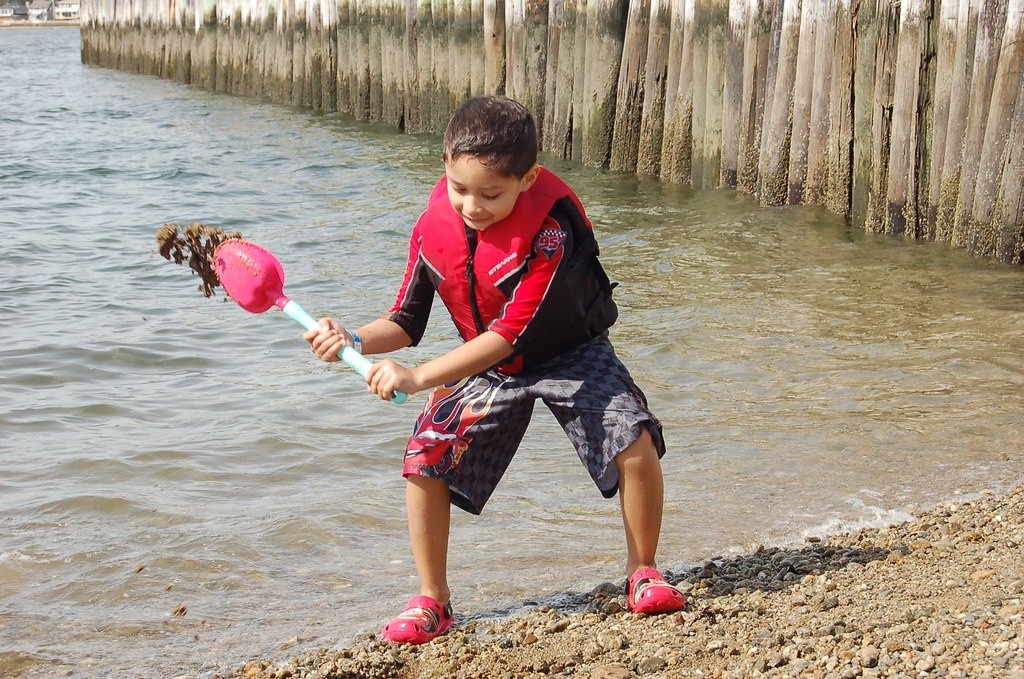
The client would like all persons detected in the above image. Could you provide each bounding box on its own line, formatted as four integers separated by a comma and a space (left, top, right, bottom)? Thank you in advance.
300, 97, 686, 646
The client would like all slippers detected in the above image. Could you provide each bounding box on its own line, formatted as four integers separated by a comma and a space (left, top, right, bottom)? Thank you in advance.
625, 568, 685, 612
382, 595, 454, 644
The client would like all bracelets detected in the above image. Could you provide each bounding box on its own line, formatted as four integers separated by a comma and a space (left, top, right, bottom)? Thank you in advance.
346, 329, 362, 354
